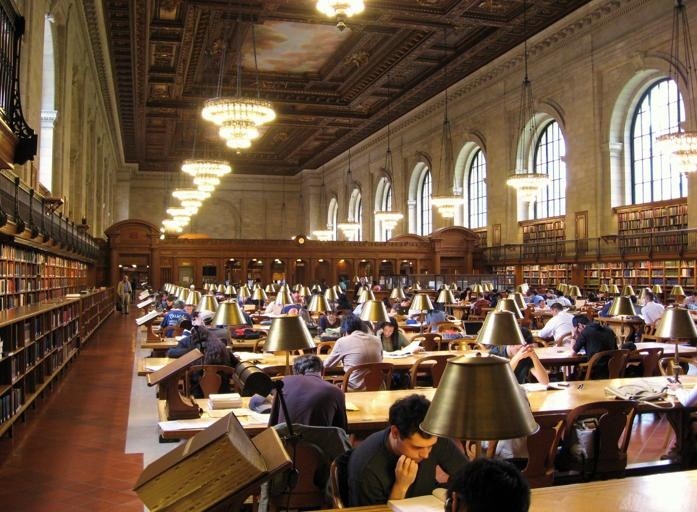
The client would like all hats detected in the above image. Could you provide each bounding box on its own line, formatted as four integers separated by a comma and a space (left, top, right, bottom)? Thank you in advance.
249, 394, 273, 413
620, 342, 635, 354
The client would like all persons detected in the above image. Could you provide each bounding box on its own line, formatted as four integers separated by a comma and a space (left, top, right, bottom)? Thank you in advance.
267, 354, 349, 438
347, 394, 471, 508
116, 274, 132, 316
444, 458, 531, 511
130, 267, 697, 394
667, 373, 696, 409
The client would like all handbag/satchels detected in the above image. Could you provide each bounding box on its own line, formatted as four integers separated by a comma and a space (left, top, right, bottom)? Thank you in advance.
603, 380, 667, 402
443, 329, 462, 339
230, 327, 262, 340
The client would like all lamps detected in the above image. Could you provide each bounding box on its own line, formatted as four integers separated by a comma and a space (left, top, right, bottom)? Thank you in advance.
158, 0, 278, 238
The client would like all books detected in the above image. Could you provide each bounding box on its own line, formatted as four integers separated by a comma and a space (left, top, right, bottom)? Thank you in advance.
205, 392, 244, 410
344, 402, 361, 412
131, 410, 269, 512
156, 420, 216, 433
0, 235, 116, 437
618, 201, 688, 259
520, 219, 566, 264
386, 486, 450, 511
205, 406, 272, 426
474, 256, 697, 299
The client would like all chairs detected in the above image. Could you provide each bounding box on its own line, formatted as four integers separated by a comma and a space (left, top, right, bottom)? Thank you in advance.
473, 197, 697, 296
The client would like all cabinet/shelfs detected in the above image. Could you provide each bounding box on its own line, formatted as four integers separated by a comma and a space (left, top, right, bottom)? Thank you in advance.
0, 214, 115, 438
